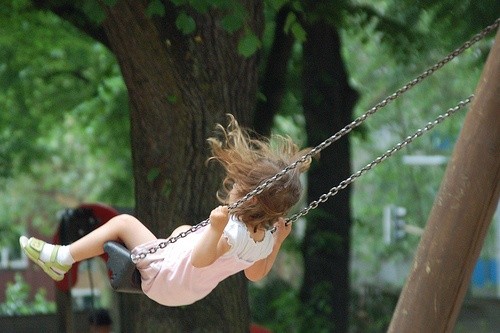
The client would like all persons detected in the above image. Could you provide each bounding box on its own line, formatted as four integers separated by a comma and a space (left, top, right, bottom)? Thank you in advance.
19, 113, 320, 307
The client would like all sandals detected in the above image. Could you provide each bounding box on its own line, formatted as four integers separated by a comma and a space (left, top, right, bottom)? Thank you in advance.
19, 235, 72, 281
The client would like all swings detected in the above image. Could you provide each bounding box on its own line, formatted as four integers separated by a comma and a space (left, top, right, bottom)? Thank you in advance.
102, 18, 500, 293
